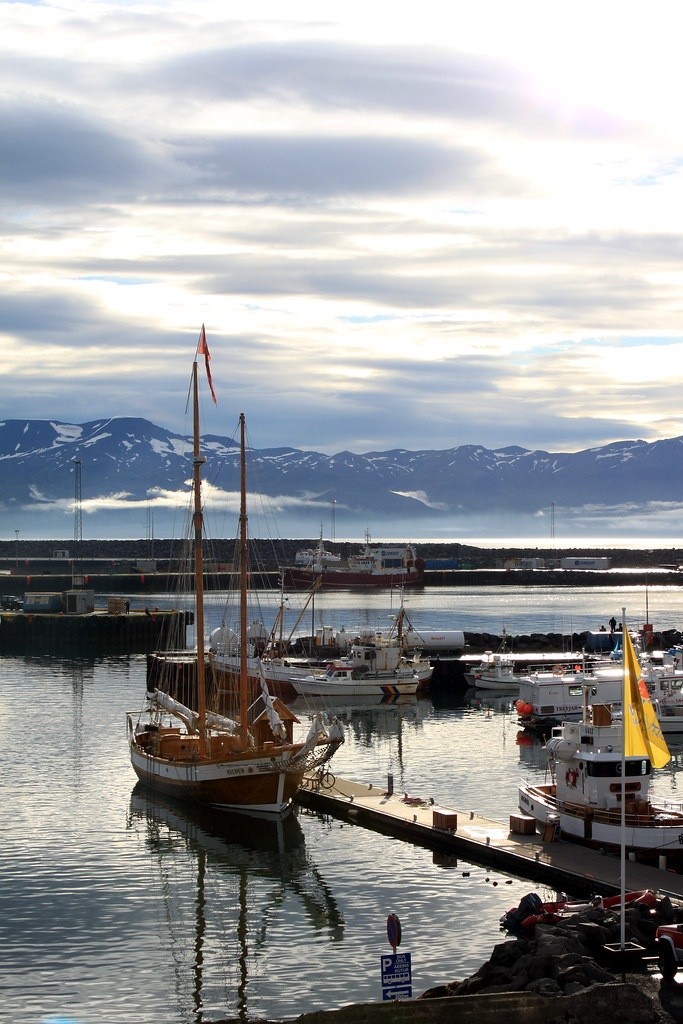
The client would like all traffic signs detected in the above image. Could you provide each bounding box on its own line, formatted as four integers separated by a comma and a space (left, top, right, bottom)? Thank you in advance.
380, 953, 412, 1001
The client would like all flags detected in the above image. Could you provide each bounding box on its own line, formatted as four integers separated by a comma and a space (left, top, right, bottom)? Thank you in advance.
198, 324, 216, 404
624, 625, 672, 769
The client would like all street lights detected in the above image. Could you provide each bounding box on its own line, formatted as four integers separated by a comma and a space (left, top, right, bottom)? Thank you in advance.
15, 530, 19, 569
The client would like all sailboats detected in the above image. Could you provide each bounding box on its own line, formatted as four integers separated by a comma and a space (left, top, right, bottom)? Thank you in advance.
384, 596, 434, 682
464, 628, 532, 689
129, 779, 309, 1022
125, 365, 343, 814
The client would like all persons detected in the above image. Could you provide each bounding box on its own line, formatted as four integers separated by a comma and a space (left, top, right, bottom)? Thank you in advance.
609, 617, 617, 632
124, 600, 131, 615
615, 623, 623, 632
599, 626, 606, 632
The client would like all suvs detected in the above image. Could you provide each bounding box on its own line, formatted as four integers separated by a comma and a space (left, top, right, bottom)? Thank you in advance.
1, 596, 24, 611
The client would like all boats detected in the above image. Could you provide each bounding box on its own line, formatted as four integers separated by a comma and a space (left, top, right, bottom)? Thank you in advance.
655, 923, 682, 960
211, 583, 683, 737
497, 886, 659, 937
278, 521, 425, 593
516, 672, 682, 864
289, 629, 420, 694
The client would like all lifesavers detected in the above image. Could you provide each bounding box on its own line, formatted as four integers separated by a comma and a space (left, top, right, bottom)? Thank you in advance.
553, 665, 563, 675
565, 767, 576, 788
